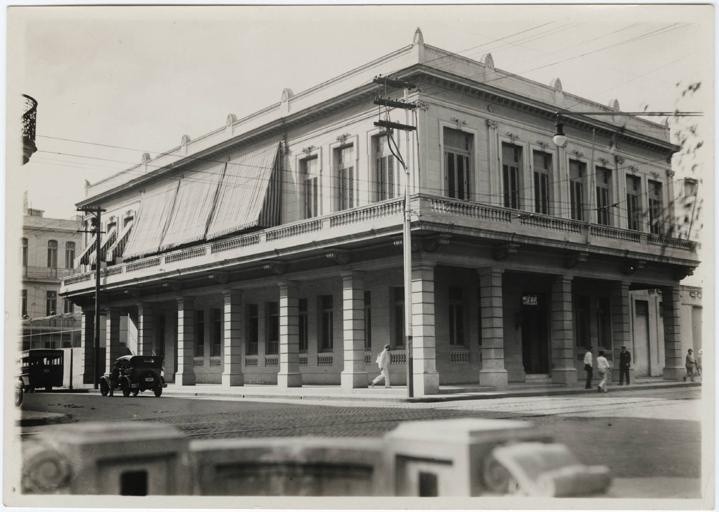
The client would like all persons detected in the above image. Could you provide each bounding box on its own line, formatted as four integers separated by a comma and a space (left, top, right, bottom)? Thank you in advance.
618, 345, 632, 387
583, 345, 596, 389
682, 349, 699, 381
595, 350, 611, 394
371, 343, 394, 388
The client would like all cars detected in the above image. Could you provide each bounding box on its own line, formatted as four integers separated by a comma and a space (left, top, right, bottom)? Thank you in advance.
99, 355, 167, 398
21, 349, 64, 393
